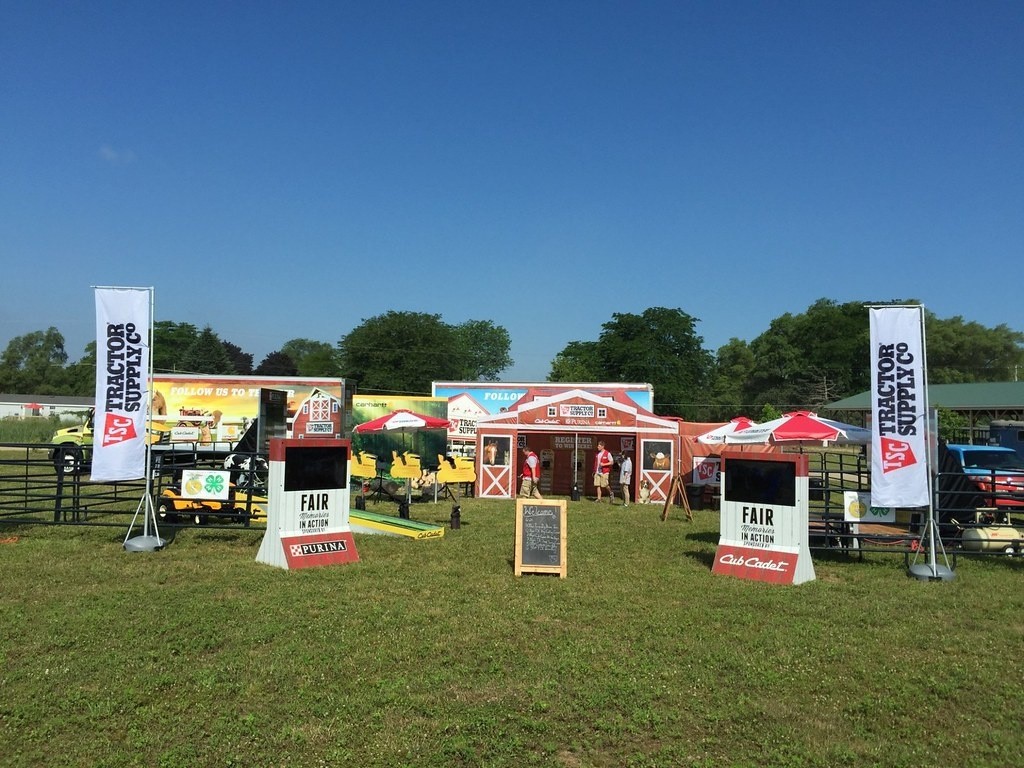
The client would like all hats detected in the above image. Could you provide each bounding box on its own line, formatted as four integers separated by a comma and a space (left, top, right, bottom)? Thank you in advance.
656, 452, 665, 459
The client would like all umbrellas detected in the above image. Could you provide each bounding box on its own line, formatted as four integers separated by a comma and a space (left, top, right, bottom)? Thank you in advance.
693, 410, 872, 452
352, 409, 451, 503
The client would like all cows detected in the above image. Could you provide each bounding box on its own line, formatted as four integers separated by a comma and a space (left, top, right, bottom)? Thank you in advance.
649, 452, 670, 470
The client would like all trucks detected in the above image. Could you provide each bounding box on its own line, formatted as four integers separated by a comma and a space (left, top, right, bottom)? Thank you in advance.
938, 443, 1024, 519
48, 372, 358, 477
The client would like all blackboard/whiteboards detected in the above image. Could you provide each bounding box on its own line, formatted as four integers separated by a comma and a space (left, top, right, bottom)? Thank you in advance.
515, 498, 568, 573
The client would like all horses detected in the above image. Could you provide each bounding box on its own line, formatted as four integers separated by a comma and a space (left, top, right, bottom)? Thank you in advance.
483, 440, 500, 466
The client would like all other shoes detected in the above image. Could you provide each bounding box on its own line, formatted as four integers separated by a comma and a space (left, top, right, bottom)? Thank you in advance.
609, 492, 615, 501
620, 502, 628, 507
593, 498, 602, 503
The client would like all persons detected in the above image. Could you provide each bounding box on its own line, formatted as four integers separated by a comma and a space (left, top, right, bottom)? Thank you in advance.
592, 440, 633, 506
520, 446, 543, 499
